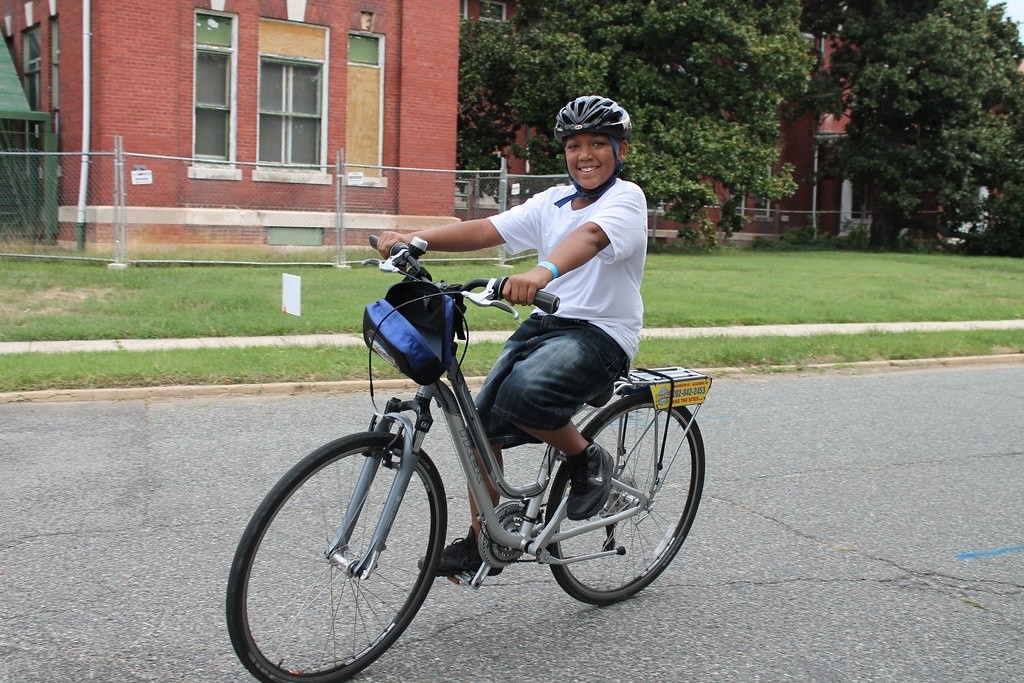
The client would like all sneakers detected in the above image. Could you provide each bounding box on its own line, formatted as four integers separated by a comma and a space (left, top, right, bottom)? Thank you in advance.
565, 435, 615, 520
418, 526, 504, 576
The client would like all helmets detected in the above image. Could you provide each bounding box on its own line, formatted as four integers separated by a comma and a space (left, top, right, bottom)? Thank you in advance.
555, 95, 633, 143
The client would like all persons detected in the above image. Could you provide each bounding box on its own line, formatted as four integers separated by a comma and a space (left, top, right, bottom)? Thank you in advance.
373, 94, 650, 579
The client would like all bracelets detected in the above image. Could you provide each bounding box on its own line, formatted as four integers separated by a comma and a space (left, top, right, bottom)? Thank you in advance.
535, 259, 559, 279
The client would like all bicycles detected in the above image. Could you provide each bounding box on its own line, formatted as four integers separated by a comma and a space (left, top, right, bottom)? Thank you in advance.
227, 236, 712, 680
